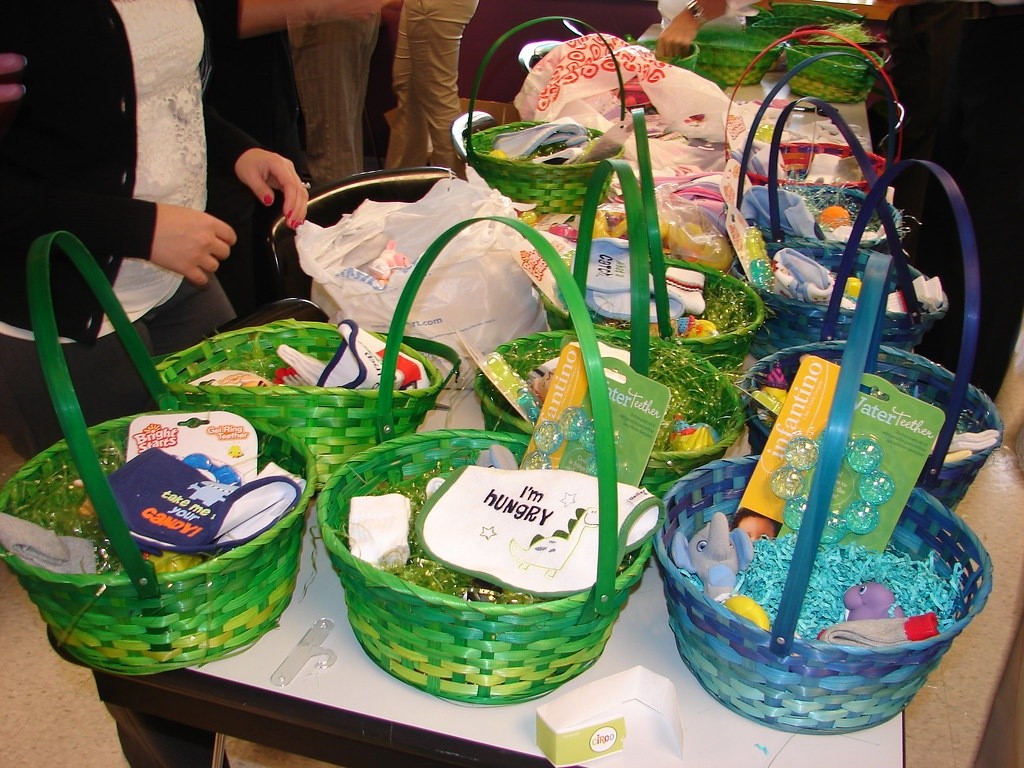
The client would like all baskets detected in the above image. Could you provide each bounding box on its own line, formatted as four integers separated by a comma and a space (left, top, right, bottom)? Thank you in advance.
153, 316, 461, 493
724, 30, 903, 194
0, 229, 316, 677
751, 15, 829, 40
651, 249, 995, 737
740, 158, 1003, 514
315, 216, 657, 706
473, 157, 743, 499
732, 95, 949, 362
785, 44, 884, 104
540, 106, 765, 376
697, 25, 785, 86
771, 2, 864, 24
733, 51, 905, 259
625, 40, 700, 75
462, 14, 628, 215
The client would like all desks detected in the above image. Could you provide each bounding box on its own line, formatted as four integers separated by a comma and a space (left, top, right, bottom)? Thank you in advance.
48, 0, 909, 766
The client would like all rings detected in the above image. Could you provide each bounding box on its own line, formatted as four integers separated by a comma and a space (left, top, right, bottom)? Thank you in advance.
299, 181, 310, 190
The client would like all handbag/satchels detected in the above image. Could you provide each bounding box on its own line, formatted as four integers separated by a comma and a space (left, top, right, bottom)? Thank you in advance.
293, 178, 552, 390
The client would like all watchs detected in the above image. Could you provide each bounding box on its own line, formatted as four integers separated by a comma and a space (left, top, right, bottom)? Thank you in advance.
687, 1, 707, 25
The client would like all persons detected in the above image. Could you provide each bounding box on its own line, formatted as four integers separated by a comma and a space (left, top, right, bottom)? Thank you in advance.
1, 0, 311, 455
656, 0, 759, 62
864, 0, 1023, 402
283, 0, 389, 193
0, 54, 30, 104
729, 506, 783, 545
382, 0, 483, 178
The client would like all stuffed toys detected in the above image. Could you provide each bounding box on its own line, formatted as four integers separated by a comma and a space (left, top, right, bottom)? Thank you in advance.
842, 580, 907, 621
672, 511, 753, 611
